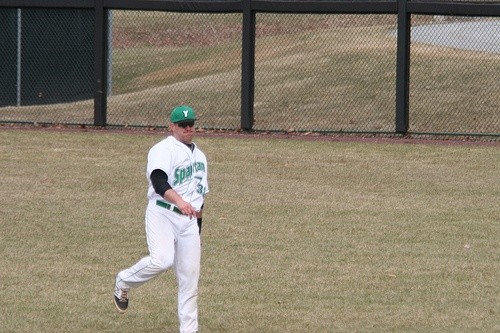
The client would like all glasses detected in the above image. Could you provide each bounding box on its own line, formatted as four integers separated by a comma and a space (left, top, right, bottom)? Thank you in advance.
175, 121, 195, 128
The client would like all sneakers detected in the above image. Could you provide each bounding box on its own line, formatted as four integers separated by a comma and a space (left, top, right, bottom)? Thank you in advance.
113, 275, 130, 313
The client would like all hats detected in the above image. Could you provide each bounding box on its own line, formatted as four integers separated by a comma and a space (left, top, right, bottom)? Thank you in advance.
170, 105, 198, 123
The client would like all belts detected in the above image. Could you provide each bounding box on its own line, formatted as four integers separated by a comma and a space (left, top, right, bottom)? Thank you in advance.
156, 200, 184, 214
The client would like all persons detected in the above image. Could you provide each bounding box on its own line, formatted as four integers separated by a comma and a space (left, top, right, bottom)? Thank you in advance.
113, 106, 210, 333
